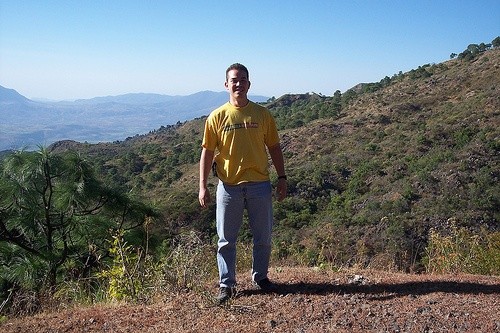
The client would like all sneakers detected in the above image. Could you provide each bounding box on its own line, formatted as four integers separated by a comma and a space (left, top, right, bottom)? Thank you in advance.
252, 278, 276, 290
217, 287, 232, 303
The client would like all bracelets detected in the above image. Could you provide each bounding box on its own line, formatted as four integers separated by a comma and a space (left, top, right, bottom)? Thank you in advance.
278, 175, 287, 181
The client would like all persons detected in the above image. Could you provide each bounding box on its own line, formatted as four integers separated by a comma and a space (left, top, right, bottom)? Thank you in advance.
199, 63, 287, 302
